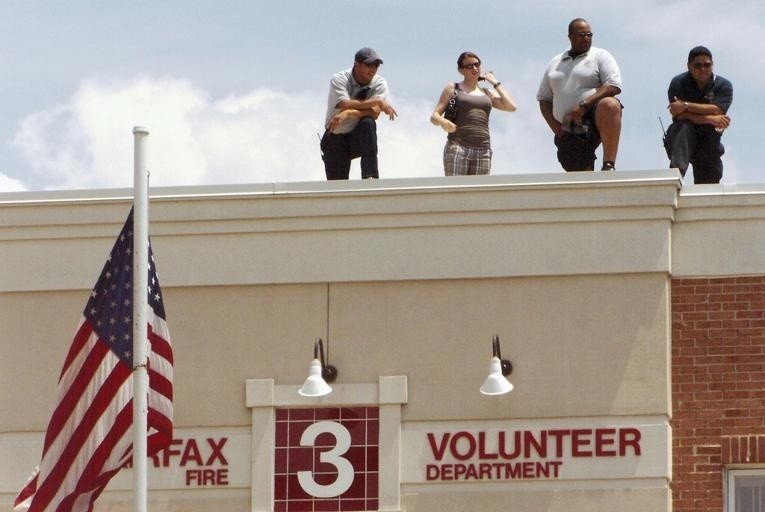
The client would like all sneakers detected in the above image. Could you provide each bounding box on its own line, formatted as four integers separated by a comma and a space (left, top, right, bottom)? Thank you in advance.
601, 161, 615, 171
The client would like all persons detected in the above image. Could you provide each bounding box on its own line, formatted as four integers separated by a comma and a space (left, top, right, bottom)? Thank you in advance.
428, 51, 518, 177
318, 46, 401, 182
665, 44, 734, 183
536, 16, 625, 173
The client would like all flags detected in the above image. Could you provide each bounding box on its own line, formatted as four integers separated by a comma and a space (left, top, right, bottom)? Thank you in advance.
8, 173, 173, 510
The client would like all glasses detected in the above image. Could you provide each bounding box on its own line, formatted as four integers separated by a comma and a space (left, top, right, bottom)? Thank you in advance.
463, 62, 479, 69
695, 62, 712, 69
574, 32, 592, 38
364, 63, 379, 68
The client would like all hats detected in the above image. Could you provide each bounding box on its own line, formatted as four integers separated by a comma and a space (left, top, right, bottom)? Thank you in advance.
355, 48, 383, 65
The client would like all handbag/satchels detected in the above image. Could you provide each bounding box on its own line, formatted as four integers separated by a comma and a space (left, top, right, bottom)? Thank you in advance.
445, 102, 456, 123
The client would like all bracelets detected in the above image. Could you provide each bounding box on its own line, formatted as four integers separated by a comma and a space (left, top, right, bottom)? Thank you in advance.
579, 99, 589, 110
684, 100, 689, 112
493, 82, 501, 89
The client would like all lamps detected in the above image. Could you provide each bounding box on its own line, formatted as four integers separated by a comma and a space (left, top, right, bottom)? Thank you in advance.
479, 333, 516, 395
294, 334, 338, 397
